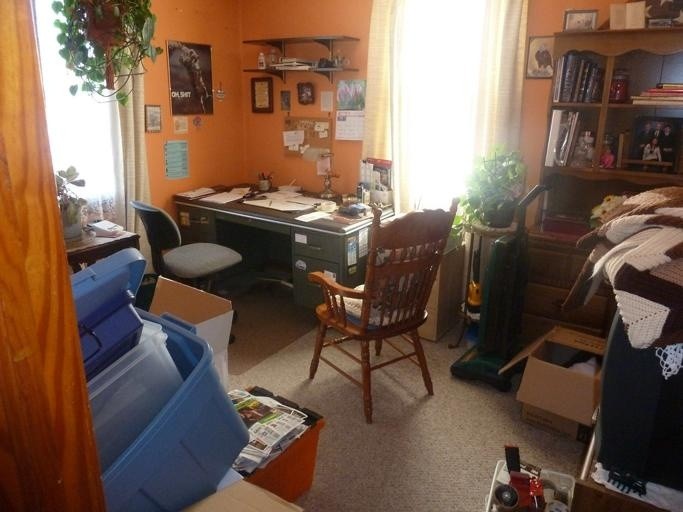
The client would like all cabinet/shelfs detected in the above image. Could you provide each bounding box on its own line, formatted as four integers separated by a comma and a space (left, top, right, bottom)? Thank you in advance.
569, 419, 683, 510
243, 35, 359, 78
516, 27, 681, 347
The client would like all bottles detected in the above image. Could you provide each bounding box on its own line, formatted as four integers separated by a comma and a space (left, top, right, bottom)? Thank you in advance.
256, 48, 280, 69
609, 66, 631, 103
334, 48, 343, 67
528, 476, 546, 512
362, 183, 370, 205
571, 130, 617, 169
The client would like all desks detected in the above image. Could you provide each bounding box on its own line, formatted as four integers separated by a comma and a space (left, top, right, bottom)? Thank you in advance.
65, 224, 140, 278
166, 182, 398, 322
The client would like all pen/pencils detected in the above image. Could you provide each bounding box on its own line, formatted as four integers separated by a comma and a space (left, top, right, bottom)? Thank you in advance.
258, 171, 273, 179
269, 200, 272, 206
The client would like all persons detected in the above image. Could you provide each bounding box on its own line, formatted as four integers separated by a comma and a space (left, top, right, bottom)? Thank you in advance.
636, 122, 675, 163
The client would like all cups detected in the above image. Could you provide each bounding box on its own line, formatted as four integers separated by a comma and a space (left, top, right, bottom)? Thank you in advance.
254, 179, 272, 192
321, 201, 336, 212
80, 230, 96, 243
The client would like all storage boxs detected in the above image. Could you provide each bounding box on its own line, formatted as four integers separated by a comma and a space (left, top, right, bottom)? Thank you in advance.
182, 475, 306, 511
70, 247, 250, 510
484, 456, 576, 512
407, 240, 464, 342
236, 385, 324, 502
497, 323, 610, 443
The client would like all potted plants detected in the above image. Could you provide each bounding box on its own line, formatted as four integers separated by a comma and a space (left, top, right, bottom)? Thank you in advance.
442, 142, 528, 227
52, 163, 88, 244
53, 0, 161, 103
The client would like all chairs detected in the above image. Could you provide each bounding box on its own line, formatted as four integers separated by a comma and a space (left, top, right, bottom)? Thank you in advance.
299, 194, 465, 426
124, 197, 242, 344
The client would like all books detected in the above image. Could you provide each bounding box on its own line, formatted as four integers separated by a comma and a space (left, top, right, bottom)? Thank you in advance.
552, 52, 603, 105
173, 187, 216, 201
629, 84, 683, 105
544, 111, 582, 167
270, 57, 315, 71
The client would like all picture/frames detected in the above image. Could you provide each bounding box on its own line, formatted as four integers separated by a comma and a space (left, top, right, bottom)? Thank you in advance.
560, 8, 599, 30
297, 81, 314, 105
249, 76, 274, 114
524, 35, 553, 79
626, 112, 682, 173
143, 104, 163, 133
